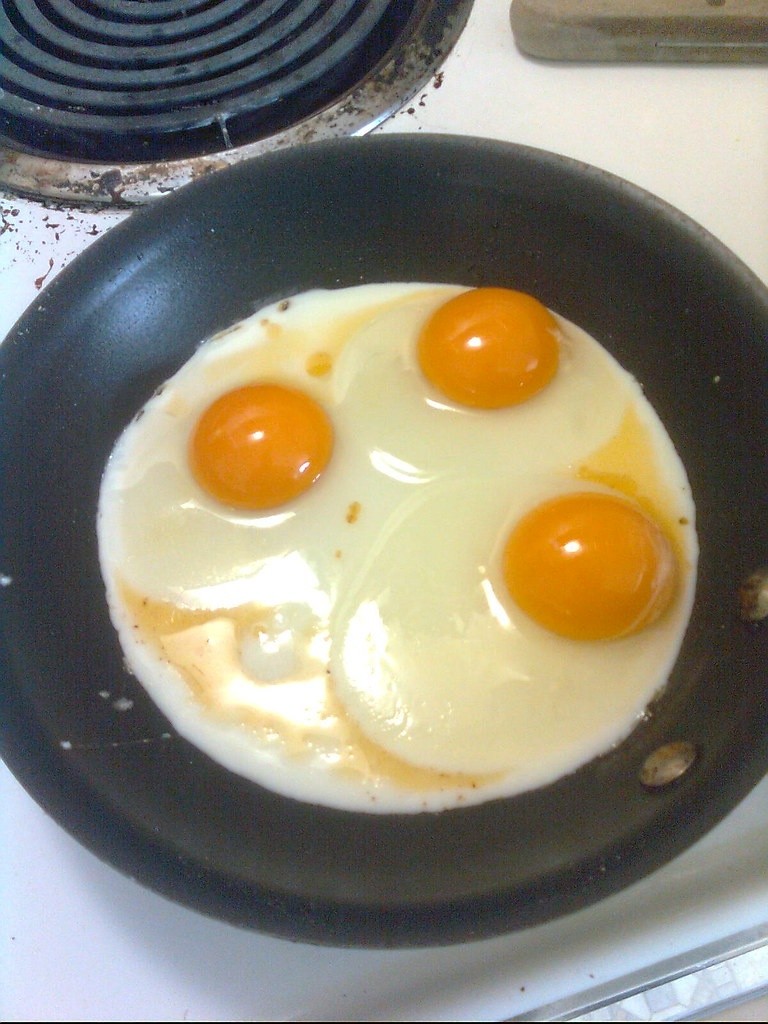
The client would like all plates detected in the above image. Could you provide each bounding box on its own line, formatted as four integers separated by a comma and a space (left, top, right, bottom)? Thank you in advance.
0, 132, 768, 949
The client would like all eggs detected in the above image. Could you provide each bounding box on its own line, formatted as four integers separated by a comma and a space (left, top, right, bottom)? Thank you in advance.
98, 281, 703, 813
